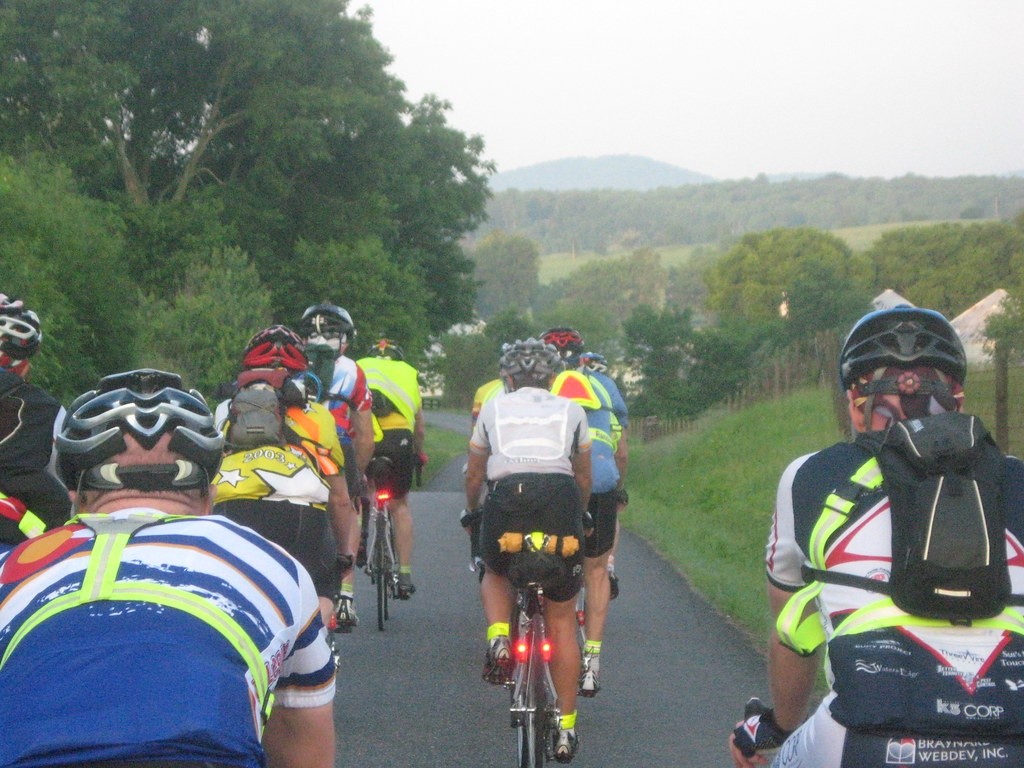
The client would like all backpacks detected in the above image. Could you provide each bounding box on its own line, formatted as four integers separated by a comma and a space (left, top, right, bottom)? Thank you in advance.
221, 386, 321, 472
305, 342, 356, 411
801, 411, 1024, 620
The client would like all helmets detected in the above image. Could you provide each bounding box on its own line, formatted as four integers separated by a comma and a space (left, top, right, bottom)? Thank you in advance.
300, 303, 354, 342
55, 368, 225, 491
367, 340, 403, 360
580, 351, 608, 373
838, 304, 967, 391
497, 337, 567, 377
538, 328, 584, 354
0, 293, 43, 359
242, 325, 308, 370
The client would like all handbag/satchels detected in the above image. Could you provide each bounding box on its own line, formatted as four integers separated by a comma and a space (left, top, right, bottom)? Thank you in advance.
369, 388, 404, 418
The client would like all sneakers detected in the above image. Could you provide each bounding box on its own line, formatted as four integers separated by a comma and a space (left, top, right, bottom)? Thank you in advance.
482, 635, 513, 684
334, 595, 360, 627
551, 727, 580, 763
578, 669, 600, 696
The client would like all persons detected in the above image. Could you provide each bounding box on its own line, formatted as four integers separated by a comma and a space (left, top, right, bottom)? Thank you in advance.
0, 287, 75, 550
194, 299, 375, 667
0, 367, 336, 768
464, 323, 630, 751
356, 339, 424, 596
729, 304, 1024, 768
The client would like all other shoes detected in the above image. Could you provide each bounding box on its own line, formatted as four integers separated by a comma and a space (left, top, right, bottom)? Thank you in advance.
398, 574, 416, 593
608, 567, 619, 600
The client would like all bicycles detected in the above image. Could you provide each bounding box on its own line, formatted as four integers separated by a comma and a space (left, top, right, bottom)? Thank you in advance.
363, 452, 429, 631
461, 508, 585, 768
